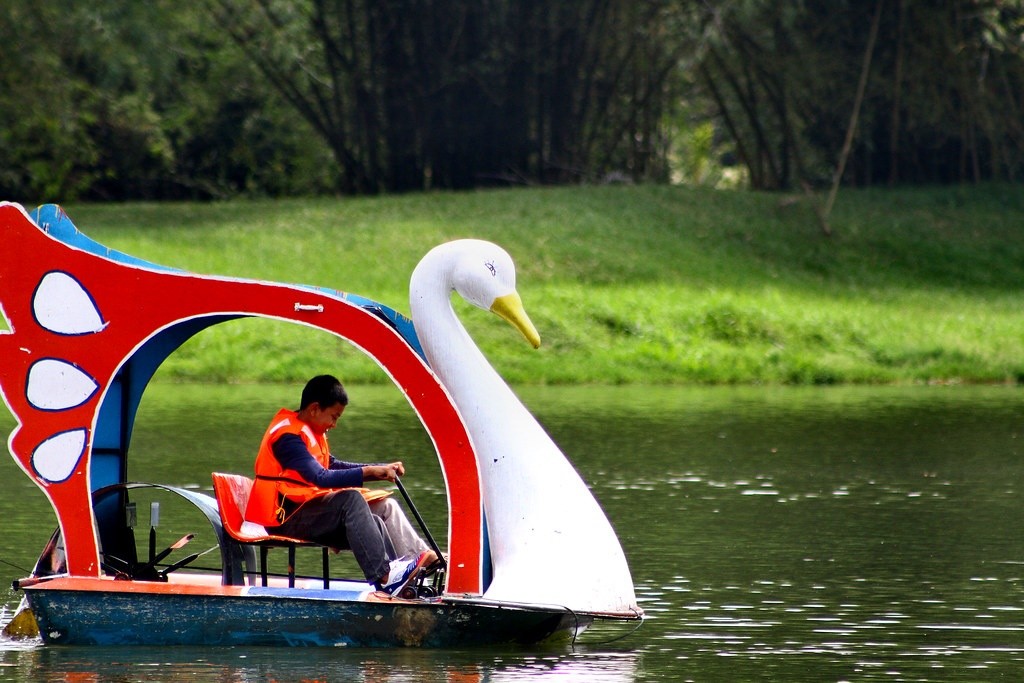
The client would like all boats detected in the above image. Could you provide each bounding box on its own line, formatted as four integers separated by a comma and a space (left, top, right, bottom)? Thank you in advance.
0, 199, 646, 650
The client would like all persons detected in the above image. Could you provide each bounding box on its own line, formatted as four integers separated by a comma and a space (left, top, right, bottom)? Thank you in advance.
246, 375, 448, 597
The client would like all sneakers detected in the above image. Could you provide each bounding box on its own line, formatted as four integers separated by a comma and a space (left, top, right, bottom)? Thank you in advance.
378, 551, 449, 598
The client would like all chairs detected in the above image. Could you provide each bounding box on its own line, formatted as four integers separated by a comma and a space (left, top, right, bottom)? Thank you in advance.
212, 472, 330, 589
